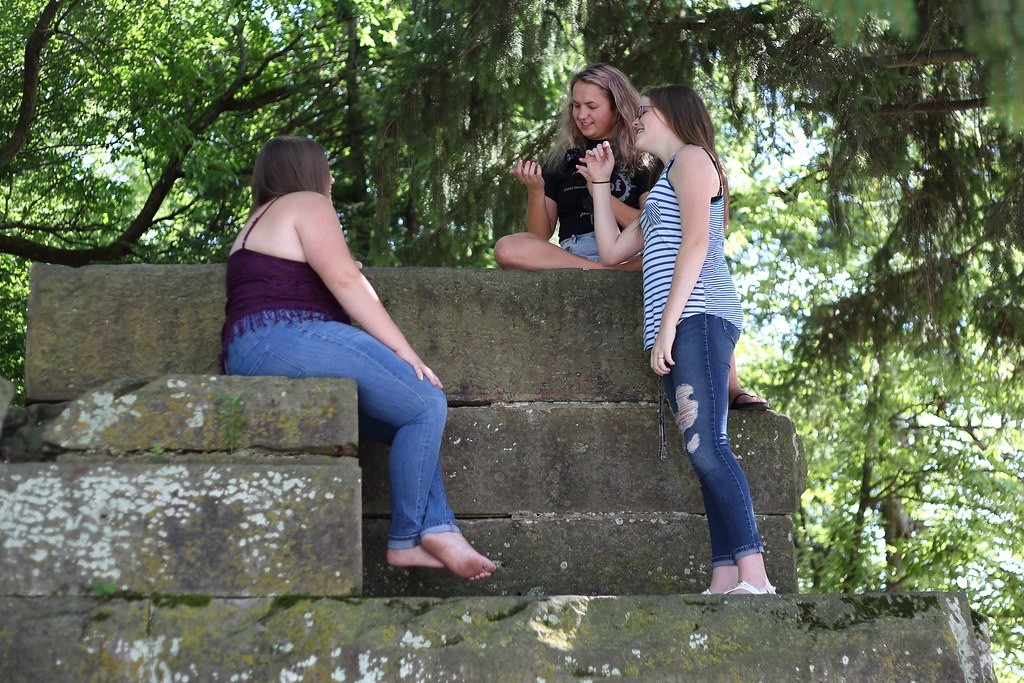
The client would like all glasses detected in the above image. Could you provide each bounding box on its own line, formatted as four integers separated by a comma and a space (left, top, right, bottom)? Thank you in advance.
634, 105, 658, 117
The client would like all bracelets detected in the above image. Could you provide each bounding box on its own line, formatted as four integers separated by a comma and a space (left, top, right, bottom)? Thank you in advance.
592, 181, 611, 184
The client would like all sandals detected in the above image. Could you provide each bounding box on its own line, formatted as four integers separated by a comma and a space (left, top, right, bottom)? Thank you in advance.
701, 589, 711, 594
723, 580, 777, 595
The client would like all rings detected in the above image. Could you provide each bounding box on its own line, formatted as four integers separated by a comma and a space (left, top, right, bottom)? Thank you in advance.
657, 356, 665, 359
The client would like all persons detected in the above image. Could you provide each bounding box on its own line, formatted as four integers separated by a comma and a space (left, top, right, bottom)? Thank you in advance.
493, 63, 771, 411
586, 86, 776, 594
217, 136, 497, 582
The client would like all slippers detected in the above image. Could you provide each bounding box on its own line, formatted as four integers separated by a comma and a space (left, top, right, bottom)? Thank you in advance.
730, 393, 770, 410
